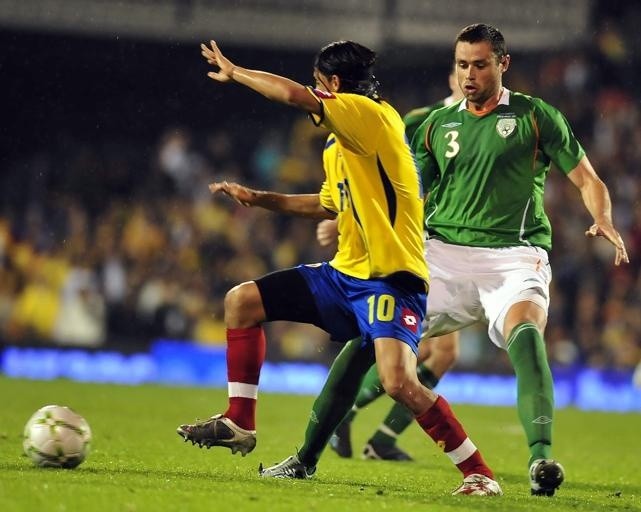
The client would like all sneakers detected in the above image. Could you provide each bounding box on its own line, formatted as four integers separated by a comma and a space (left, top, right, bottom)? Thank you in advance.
529, 458, 564, 495
450, 473, 503, 497
328, 420, 412, 460
259, 456, 316, 480
176, 414, 256, 456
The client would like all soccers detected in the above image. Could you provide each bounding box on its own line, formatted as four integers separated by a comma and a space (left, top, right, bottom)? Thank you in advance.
23, 404, 92, 468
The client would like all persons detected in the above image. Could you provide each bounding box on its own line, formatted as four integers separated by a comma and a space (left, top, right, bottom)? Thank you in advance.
172, 38, 506, 500
258, 23, 632, 496
330, 324, 463, 465
1, 2, 640, 379
